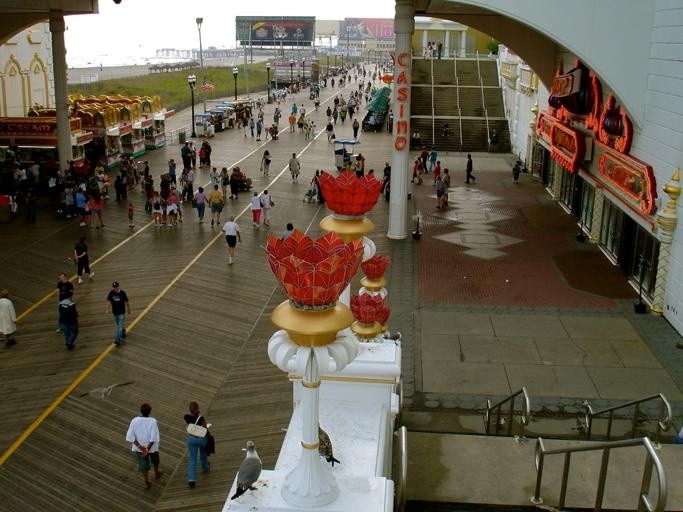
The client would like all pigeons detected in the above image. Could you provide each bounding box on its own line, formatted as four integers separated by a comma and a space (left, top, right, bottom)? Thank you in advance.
228, 440, 264, 501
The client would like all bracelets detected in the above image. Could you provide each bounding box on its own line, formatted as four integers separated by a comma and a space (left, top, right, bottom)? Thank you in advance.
138, 445, 140, 448
145, 448, 148, 451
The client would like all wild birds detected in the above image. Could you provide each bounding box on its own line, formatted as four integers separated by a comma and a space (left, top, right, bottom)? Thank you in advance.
315, 424, 340, 469
80, 379, 139, 399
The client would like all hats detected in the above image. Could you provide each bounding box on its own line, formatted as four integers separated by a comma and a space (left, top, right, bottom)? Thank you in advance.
112, 281, 119, 288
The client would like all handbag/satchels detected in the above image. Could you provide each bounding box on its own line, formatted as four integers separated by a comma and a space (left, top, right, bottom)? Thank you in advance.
260, 202, 264, 207
270, 201, 275, 207
331, 133, 337, 139
187, 424, 207, 438
266, 158, 271, 164
223, 180, 230, 185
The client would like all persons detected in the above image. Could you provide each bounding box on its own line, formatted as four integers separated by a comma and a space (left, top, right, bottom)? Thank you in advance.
427, 42, 432, 59
184, 402, 210, 486
0, 289, 17, 346
513, 161, 521, 183
105, 282, 128, 345
437, 42, 442, 59
74, 237, 95, 284
59, 292, 80, 350
56, 272, 74, 333
125, 404, 162, 489
464, 154, 475, 183
490, 128, 497, 151
432, 42, 438, 57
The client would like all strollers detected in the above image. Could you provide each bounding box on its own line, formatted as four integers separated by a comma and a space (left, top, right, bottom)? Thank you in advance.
299, 181, 317, 205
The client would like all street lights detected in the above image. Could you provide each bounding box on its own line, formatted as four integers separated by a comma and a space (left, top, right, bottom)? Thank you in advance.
264, 63, 272, 103
195, 17, 205, 71
367, 49, 377, 65
325, 48, 350, 75
186, 74, 199, 138
287, 59, 293, 94
230, 66, 238, 100
301, 57, 305, 76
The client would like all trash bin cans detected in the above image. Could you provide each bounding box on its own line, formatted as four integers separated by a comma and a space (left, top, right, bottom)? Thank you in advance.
179, 131, 186, 144
268, 95, 273, 104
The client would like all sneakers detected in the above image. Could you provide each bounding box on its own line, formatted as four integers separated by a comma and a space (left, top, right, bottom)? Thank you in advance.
78, 278, 83, 283
153, 219, 184, 228
142, 463, 211, 491
89, 272, 94, 278
252, 221, 272, 228
243, 134, 263, 142
